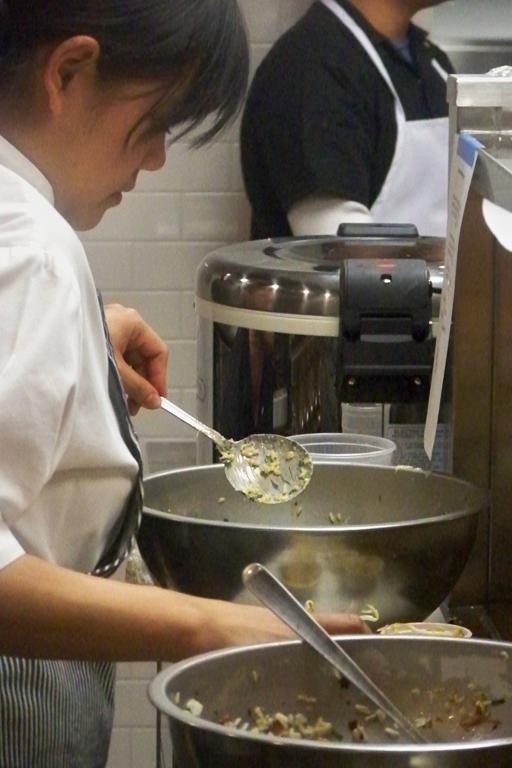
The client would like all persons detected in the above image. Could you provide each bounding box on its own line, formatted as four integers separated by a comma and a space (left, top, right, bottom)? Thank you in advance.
2, 1, 373, 768
241, 3, 457, 242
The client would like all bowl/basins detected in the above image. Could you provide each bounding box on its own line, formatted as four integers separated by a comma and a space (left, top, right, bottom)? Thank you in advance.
143, 633, 511, 766
132, 461, 486, 631
285, 432, 397, 465
